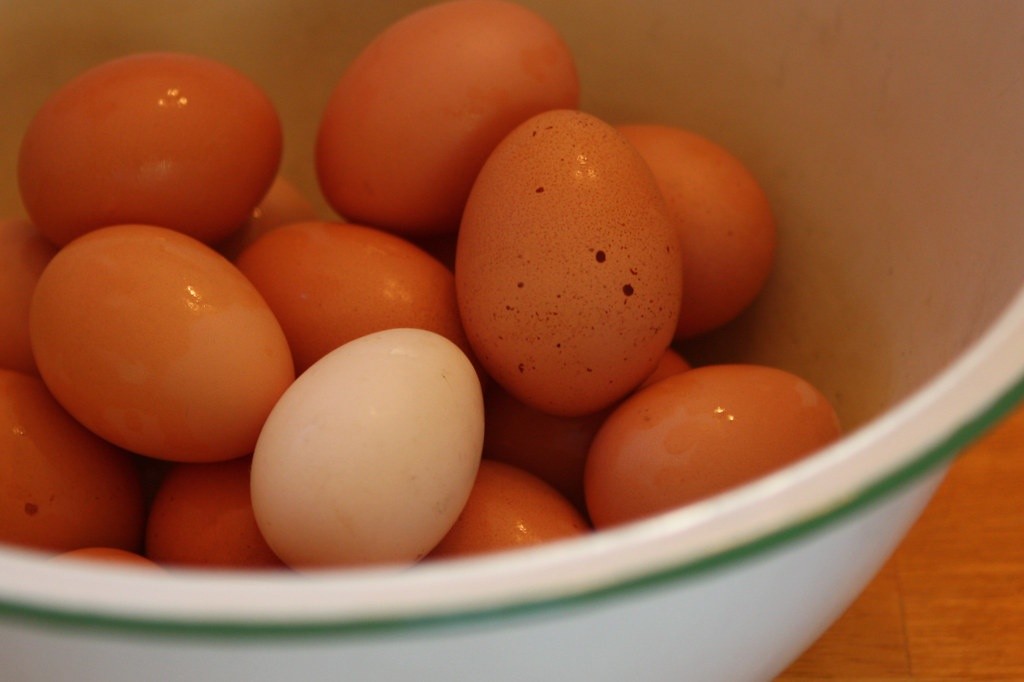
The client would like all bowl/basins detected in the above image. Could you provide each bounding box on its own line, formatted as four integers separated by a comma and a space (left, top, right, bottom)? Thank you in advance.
0, 0, 1024, 682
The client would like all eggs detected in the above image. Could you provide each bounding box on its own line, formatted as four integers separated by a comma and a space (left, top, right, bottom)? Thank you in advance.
0, 0, 842, 576
